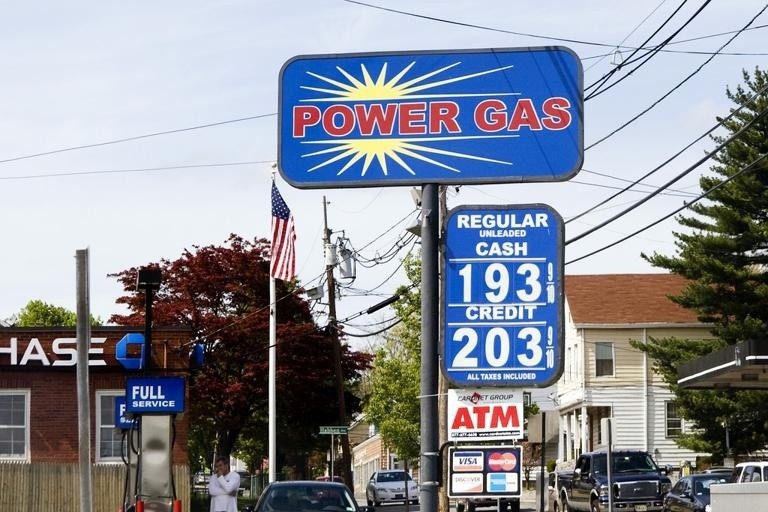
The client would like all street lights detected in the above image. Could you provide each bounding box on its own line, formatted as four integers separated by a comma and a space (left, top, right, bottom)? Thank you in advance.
136, 267, 162, 374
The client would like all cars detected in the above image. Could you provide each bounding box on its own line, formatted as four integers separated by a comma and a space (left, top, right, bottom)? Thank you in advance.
549, 471, 575, 512
662, 461, 768, 512
454, 495, 520, 512
244, 479, 376, 512
309, 475, 345, 502
366, 469, 419, 507
192, 468, 251, 491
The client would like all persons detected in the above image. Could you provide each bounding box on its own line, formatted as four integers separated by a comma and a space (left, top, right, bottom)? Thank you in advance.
207, 458, 240, 512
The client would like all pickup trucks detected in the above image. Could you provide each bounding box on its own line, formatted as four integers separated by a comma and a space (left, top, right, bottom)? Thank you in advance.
556, 447, 673, 511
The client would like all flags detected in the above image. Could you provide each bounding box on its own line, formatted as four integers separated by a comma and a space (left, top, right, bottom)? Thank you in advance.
268, 178, 296, 284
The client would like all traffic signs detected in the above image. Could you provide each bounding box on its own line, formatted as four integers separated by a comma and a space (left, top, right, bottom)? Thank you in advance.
317, 426, 347, 434
